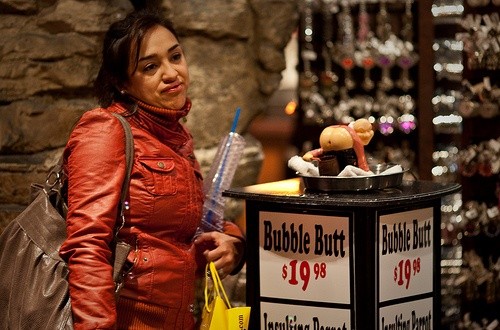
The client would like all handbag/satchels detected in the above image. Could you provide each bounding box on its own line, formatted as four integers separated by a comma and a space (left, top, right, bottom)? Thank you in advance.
0, 109, 135, 330
200, 261, 252, 330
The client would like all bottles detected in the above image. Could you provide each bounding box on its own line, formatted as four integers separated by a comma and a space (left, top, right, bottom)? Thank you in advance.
194, 131, 246, 250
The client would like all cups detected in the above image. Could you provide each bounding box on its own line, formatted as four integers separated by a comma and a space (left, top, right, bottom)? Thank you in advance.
318, 155, 339, 176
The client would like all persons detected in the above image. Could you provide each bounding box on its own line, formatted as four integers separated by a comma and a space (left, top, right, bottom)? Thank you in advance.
57, 10, 248, 330
